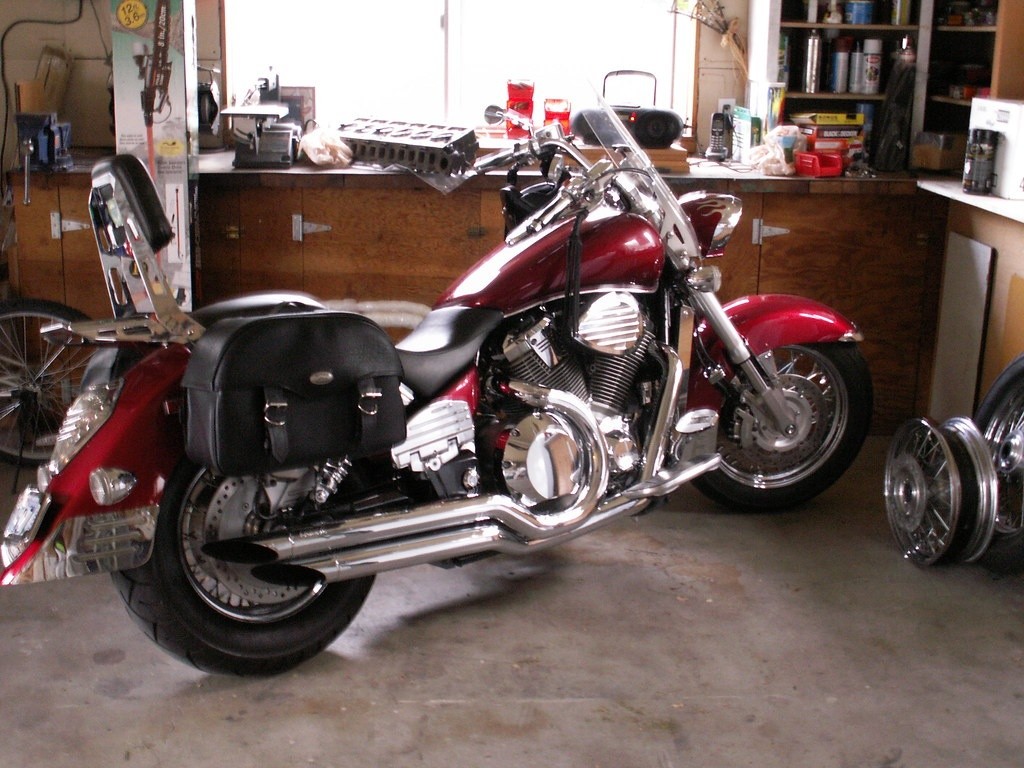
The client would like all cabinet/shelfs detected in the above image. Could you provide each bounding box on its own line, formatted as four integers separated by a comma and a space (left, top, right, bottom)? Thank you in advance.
4, 143, 946, 436
924, 0, 1024, 178
747, 0, 920, 169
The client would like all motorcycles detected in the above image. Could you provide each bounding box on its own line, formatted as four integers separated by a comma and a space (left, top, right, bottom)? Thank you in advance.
0, 87, 876, 680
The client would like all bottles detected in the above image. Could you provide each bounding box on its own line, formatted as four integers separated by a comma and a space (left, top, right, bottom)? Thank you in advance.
778, 0, 917, 96
961, 127, 1000, 194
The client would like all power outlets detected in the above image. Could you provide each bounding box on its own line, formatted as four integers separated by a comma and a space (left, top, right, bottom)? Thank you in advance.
718, 98, 736, 116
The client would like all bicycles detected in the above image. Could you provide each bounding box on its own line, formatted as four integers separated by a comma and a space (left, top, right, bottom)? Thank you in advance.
0, 297, 97, 494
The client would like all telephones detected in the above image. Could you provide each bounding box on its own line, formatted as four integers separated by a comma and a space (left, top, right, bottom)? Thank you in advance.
710, 113, 725, 153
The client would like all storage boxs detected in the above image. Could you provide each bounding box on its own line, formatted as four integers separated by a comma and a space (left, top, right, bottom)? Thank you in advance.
732, 105, 760, 156
912, 130, 966, 170
782, 107, 865, 162
966, 97, 1024, 200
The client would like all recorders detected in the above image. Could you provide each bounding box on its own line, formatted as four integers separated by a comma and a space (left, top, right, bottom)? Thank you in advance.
570, 69, 683, 150
